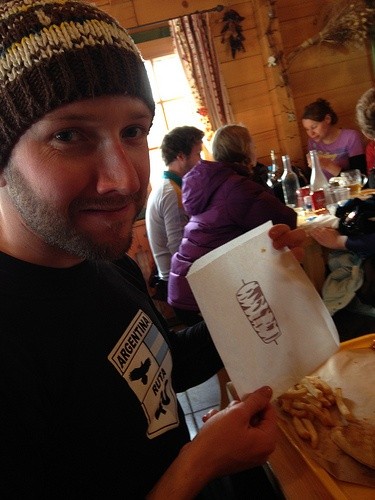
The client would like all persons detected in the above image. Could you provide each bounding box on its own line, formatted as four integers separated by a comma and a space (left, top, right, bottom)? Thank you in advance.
167, 124, 299, 309
301, 98, 369, 189
310, 88, 375, 256
0, 0, 303, 500
145, 127, 206, 281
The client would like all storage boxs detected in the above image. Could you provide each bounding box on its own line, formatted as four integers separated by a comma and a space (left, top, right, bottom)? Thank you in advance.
276, 334, 375, 500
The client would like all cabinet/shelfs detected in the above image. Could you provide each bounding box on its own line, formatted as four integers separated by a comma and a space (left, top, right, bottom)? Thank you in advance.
128, 220, 160, 292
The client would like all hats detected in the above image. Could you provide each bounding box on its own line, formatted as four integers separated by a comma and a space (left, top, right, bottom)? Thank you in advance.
0, 0, 156, 166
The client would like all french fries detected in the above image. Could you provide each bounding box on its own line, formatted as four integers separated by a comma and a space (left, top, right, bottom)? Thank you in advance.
278, 377, 352, 447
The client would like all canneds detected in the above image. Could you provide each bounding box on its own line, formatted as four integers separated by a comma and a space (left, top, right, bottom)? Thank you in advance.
296, 186, 311, 207
310, 189, 326, 211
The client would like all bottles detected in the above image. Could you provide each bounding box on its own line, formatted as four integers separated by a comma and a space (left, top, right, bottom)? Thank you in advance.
281, 155, 304, 212
310, 150, 333, 208
296, 169, 308, 187
270, 151, 286, 205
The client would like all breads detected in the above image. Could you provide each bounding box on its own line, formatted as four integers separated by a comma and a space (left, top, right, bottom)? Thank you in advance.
314, 416, 375, 486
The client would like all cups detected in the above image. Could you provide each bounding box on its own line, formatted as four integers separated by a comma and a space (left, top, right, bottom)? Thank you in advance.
323, 169, 369, 209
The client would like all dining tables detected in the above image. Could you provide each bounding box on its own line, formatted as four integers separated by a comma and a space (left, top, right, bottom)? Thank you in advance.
225, 380, 335, 500
